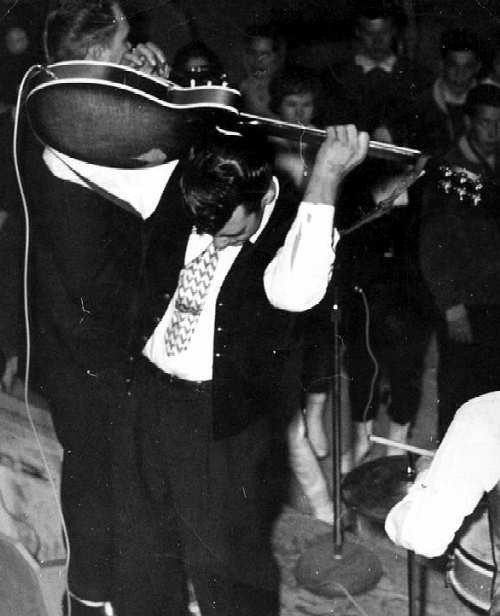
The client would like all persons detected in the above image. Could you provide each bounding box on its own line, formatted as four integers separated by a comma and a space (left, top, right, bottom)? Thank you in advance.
2, 0, 500, 537
380, 387, 500, 616
39, 39, 375, 615
15, 0, 169, 615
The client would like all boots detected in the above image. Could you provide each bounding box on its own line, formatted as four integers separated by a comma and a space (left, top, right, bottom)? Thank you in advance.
305, 389, 333, 457
336, 420, 376, 475
385, 418, 412, 457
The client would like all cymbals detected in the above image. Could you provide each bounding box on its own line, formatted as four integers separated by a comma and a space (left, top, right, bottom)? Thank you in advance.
341, 456, 429, 522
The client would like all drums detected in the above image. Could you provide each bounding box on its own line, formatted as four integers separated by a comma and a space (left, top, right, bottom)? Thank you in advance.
445, 505, 493, 612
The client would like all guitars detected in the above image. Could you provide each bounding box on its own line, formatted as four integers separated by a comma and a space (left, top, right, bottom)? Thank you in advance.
25, 60, 483, 212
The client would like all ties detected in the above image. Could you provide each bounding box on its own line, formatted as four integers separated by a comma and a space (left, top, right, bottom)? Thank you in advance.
156, 238, 226, 363
